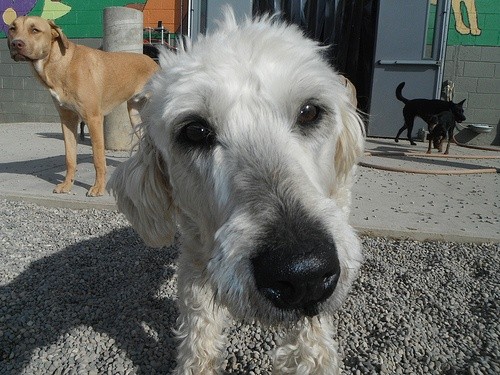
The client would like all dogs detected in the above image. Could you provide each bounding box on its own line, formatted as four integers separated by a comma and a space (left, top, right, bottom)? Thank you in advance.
104, 2, 373, 375
394, 81, 467, 155
7, 15, 162, 198
